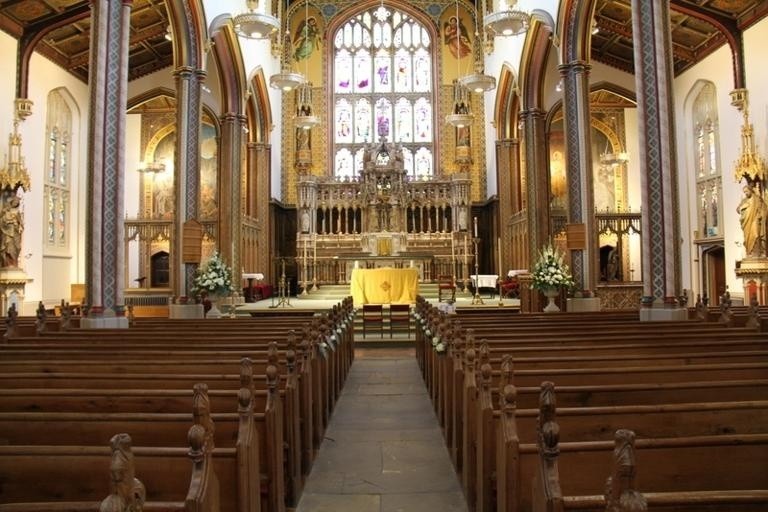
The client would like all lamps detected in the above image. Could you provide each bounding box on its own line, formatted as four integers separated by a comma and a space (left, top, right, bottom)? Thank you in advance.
445, 0, 532, 128
600, 112, 630, 177
232, 0, 319, 129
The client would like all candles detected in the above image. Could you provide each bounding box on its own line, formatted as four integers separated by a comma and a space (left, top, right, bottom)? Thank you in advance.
282, 259, 286, 274
303, 233, 316, 267
498, 238, 502, 276
451, 230, 468, 264
474, 217, 478, 237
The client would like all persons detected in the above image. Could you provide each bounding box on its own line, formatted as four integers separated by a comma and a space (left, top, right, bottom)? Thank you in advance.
0, 199, 26, 268
292, 16, 323, 62
443, 15, 472, 59
736, 185, 768, 259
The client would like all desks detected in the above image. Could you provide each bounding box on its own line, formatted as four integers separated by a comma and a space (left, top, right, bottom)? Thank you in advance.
242, 273, 264, 303
470, 274, 498, 299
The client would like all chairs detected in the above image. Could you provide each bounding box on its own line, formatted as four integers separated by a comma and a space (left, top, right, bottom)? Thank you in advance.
362, 304, 410, 339
439, 275, 456, 303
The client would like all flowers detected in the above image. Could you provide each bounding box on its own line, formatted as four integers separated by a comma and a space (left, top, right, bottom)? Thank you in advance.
528, 235, 576, 291
190, 249, 236, 294
